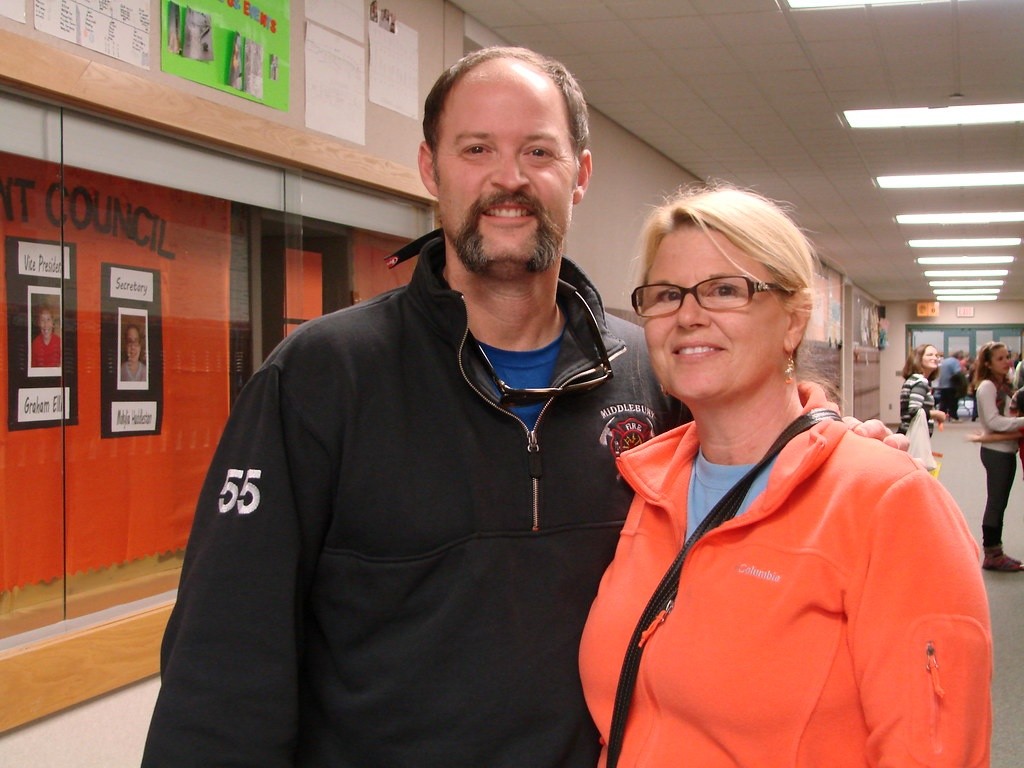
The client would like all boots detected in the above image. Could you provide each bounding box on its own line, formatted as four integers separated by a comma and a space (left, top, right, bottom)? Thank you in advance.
982, 545, 1024, 572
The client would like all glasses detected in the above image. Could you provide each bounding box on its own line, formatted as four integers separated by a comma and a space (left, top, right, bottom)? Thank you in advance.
126, 338, 140, 347
631, 276, 796, 317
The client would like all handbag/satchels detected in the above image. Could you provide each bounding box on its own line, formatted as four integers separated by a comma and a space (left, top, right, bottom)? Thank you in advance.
905, 408, 936, 471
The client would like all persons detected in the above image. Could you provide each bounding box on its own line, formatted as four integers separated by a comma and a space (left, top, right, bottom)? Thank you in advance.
121, 323, 146, 381
580, 186, 1024, 768
31, 306, 61, 368
141, 46, 925, 768
